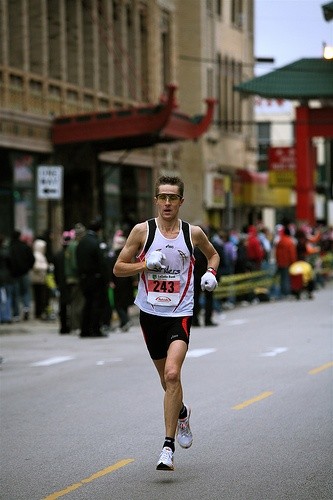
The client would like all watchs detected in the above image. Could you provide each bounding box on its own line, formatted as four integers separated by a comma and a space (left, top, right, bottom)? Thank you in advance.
207, 267, 217, 276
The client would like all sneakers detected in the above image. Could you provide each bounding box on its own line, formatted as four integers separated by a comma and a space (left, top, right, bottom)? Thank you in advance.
155, 447, 175, 471
176, 404, 194, 448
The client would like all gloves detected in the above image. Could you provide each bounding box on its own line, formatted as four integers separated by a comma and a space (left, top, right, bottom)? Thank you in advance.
146, 250, 166, 271
201, 267, 218, 291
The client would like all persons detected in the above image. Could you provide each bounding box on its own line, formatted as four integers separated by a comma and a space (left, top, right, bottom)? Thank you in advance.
194, 225, 220, 326
208, 223, 333, 312
0, 223, 135, 337
113, 175, 221, 470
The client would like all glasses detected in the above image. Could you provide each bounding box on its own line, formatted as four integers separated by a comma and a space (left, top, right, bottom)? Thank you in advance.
156, 194, 182, 204
